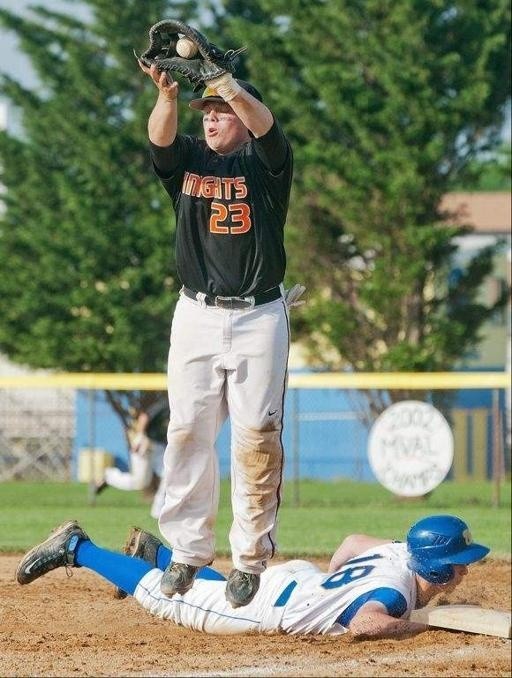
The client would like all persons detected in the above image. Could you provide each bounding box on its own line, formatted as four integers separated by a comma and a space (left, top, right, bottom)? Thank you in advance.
16, 515, 490, 637
138, 18, 294, 610
94, 393, 170, 495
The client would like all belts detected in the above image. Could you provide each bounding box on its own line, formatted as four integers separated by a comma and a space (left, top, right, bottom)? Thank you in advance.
184, 287, 280, 307
274, 580, 297, 607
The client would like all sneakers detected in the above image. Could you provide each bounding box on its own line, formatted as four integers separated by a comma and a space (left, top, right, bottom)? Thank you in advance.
226, 570, 260, 608
161, 561, 196, 597
16, 521, 89, 584
114, 527, 160, 599
96, 479, 106, 494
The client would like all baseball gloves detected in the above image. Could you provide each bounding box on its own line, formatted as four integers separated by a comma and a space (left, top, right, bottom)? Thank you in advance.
141, 20, 248, 94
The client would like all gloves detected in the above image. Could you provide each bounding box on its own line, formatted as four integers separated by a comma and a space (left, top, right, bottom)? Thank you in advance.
286, 284, 306, 308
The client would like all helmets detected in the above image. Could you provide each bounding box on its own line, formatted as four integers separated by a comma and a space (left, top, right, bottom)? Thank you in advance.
406, 516, 488, 585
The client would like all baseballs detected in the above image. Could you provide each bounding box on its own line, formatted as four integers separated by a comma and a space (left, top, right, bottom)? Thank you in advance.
176, 37, 200, 58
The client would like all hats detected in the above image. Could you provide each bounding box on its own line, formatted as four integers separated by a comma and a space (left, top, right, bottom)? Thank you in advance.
190, 80, 261, 112
